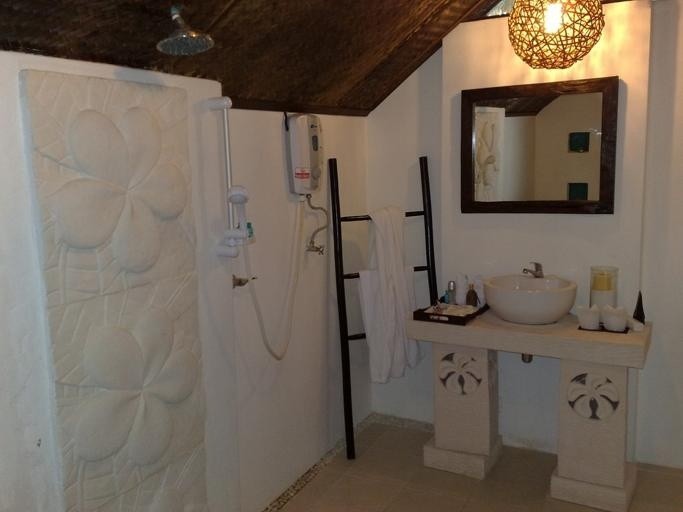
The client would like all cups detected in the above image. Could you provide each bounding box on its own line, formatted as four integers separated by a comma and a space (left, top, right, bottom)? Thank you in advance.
575, 308, 600, 330
601, 310, 628, 332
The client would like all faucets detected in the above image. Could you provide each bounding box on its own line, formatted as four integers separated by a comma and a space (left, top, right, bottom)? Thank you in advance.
523, 261, 544, 278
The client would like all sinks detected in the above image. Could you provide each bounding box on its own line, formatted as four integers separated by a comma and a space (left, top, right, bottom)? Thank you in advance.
482, 274, 577, 323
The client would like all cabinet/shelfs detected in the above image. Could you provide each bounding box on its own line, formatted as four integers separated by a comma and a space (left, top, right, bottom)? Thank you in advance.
406, 313, 653, 512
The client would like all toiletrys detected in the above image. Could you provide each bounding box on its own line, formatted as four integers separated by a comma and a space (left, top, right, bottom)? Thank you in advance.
447, 280, 456, 305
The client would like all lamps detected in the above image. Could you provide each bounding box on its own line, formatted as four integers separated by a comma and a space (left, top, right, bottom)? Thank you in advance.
508, 0, 605, 69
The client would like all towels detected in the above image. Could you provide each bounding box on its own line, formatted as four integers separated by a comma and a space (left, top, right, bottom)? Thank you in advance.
358, 204, 427, 384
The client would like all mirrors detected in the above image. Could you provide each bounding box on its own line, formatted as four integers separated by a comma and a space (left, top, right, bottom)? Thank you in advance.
461, 75, 619, 214
